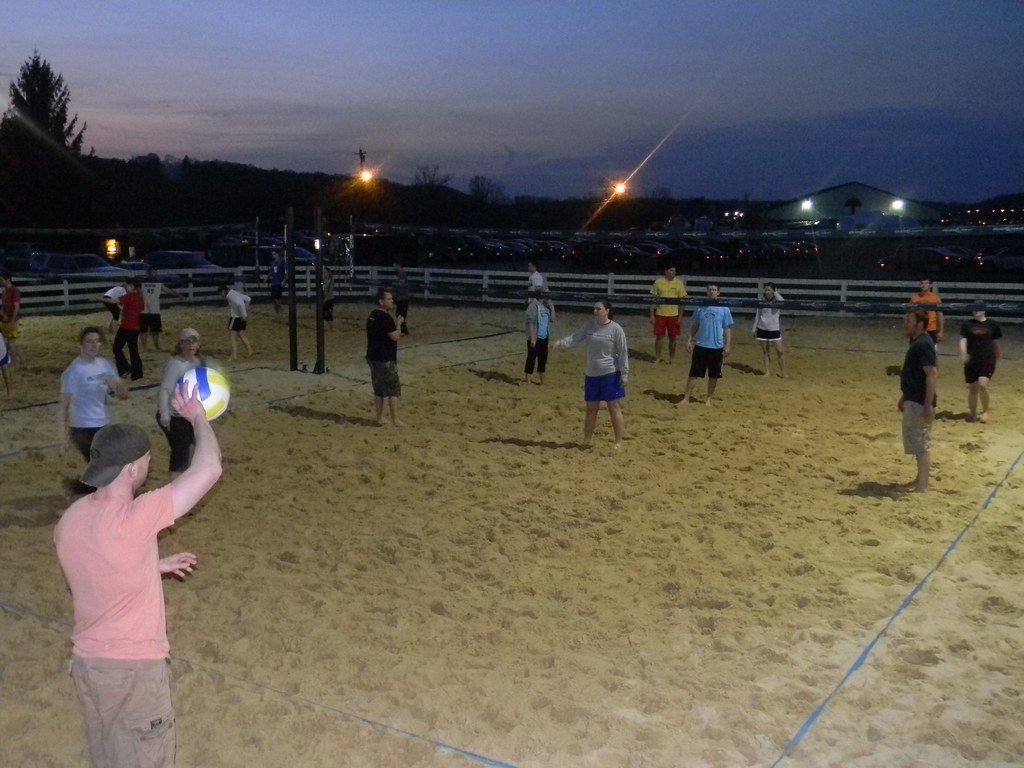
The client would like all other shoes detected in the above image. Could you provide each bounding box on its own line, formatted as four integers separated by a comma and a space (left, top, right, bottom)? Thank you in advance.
129, 374, 140, 381
119, 365, 131, 376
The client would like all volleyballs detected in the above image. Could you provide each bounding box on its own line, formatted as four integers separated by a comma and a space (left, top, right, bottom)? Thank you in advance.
177, 366, 230, 421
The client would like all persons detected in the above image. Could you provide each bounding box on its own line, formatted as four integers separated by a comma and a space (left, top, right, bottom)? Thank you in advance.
674, 281, 734, 406
548, 302, 628, 449
365, 291, 405, 425
0, 273, 20, 395
524, 288, 555, 385
649, 266, 687, 365
753, 284, 786, 377
59, 328, 128, 499
910, 280, 945, 351
528, 262, 543, 290
323, 267, 335, 326
271, 249, 288, 320
386, 264, 409, 335
156, 328, 202, 482
898, 309, 937, 493
218, 286, 253, 361
959, 301, 1003, 421
54, 379, 223, 768
94, 267, 184, 381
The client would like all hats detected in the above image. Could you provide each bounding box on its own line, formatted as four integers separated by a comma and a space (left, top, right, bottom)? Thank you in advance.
79, 422, 152, 488
179, 328, 199, 342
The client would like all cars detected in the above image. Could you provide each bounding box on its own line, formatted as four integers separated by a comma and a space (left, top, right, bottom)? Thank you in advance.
32, 251, 126, 295
137, 251, 235, 287
4, 242, 36, 270
117, 260, 184, 298
216, 218, 1024, 280
